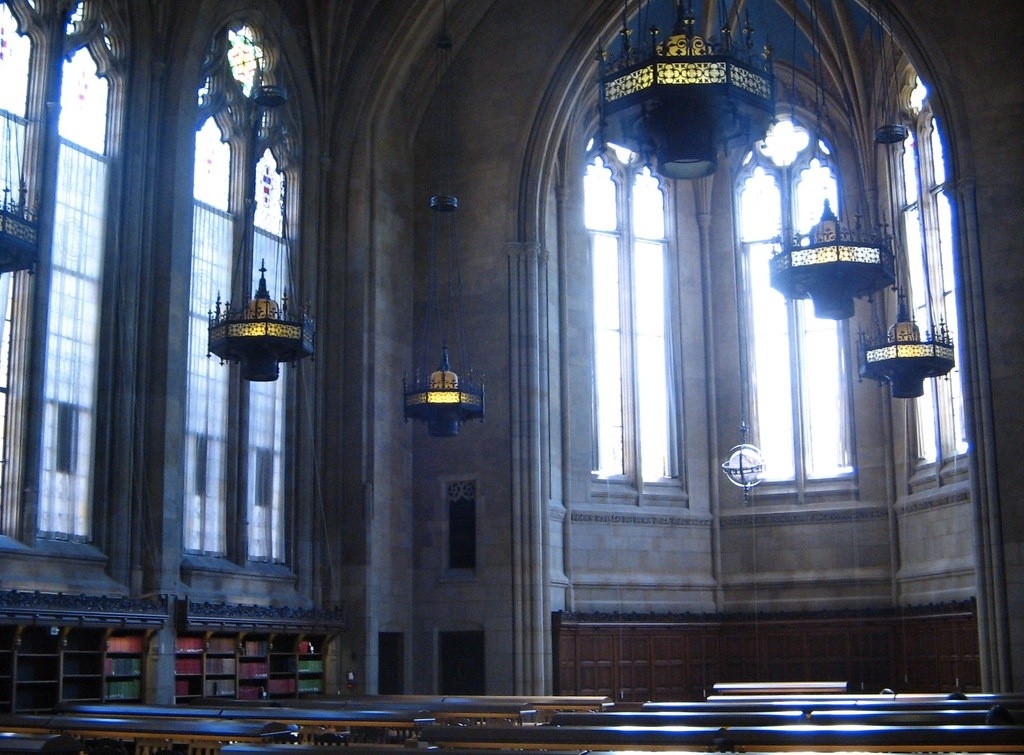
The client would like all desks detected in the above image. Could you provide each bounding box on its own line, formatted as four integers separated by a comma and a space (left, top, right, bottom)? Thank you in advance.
0, 683, 1023, 755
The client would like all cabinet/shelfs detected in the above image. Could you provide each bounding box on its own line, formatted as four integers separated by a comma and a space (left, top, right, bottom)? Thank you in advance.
2, 620, 345, 718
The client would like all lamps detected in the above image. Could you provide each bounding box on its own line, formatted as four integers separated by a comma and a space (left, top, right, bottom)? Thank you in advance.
593, 0, 776, 181
205, 0, 315, 384
859, 0, 955, 397
402, 0, 485, 436
0, 0, 67, 281
775, 0, 896, 320
